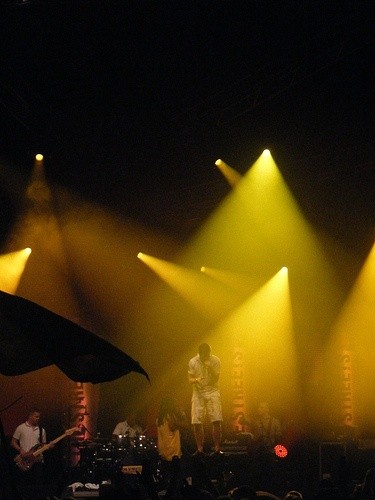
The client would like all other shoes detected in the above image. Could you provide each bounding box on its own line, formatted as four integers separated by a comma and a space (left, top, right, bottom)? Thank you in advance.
192, 450, 205, 456
210, 449, 224, 456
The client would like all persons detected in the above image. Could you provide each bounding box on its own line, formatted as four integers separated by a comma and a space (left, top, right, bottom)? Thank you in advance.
187, 343, 223, 455
12, 402, 375, 500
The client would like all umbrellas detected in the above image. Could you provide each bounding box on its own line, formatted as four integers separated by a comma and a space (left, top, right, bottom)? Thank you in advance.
0, 291, 153, 387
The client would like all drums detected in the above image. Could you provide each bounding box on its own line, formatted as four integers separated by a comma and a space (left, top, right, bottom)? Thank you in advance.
113, 434, 131, 452
100, 438, 116, 452
112, 453, 156, 490
132, 435, 158, 451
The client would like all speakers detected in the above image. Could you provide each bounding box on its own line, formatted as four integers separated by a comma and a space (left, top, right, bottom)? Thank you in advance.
318, 441, 349, 488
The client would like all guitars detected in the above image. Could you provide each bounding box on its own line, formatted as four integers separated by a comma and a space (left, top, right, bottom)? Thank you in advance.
14, 426, 82, 471
